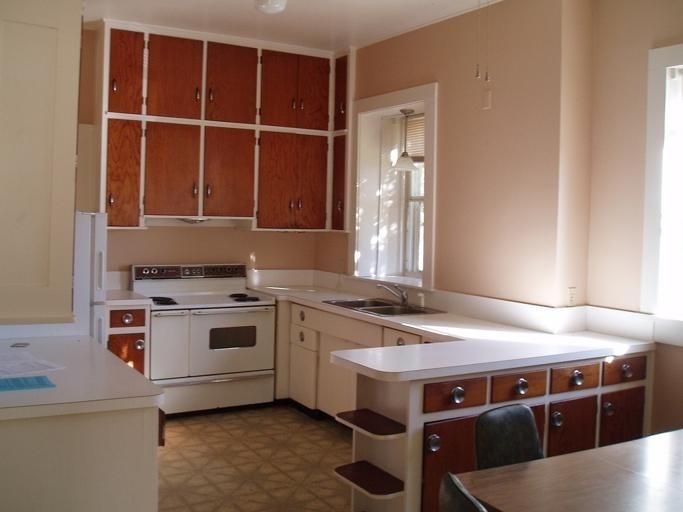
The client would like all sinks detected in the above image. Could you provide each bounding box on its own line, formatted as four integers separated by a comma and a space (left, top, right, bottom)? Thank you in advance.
334, 299, 393, 308
360, 306, 428, 316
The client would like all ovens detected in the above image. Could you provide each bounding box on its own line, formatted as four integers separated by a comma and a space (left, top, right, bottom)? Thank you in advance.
148, 311, 275, 420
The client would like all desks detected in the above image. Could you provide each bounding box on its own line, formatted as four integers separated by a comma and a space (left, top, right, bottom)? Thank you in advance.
0, 328, 160, 511
454, 421, 683, 510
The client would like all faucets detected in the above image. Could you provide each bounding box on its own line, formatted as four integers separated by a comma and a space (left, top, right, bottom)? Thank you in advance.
375, 282, 409, 306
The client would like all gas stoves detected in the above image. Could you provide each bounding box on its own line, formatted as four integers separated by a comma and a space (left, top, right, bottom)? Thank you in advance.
148, 290, 276, 310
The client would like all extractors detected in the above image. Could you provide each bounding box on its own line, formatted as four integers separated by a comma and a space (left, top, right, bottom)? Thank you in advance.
144, 216, 253, 226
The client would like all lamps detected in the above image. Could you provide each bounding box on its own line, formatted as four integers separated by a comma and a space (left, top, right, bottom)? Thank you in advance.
252, 0, 289, 16
391, 105, 420, 171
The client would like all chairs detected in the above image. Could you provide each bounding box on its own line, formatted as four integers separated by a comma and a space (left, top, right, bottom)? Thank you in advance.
441, 472, 492, 511
475, 402, 546, 469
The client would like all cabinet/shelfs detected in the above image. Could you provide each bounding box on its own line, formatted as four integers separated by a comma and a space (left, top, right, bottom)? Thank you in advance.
286, 304, 423, 420
417, 367, 549, 512
329, 361, 414, 510
332, 55, 348, 132
143, 119, 257, 221
78, 24, 145, 116
106, 287, 150, 386
546, 351, 653, 458
260, 43, 331, 132
330, 135, 347, 231
258, 129, 328, 230
146, 28, 258, 125
77, 117, 142, 227
